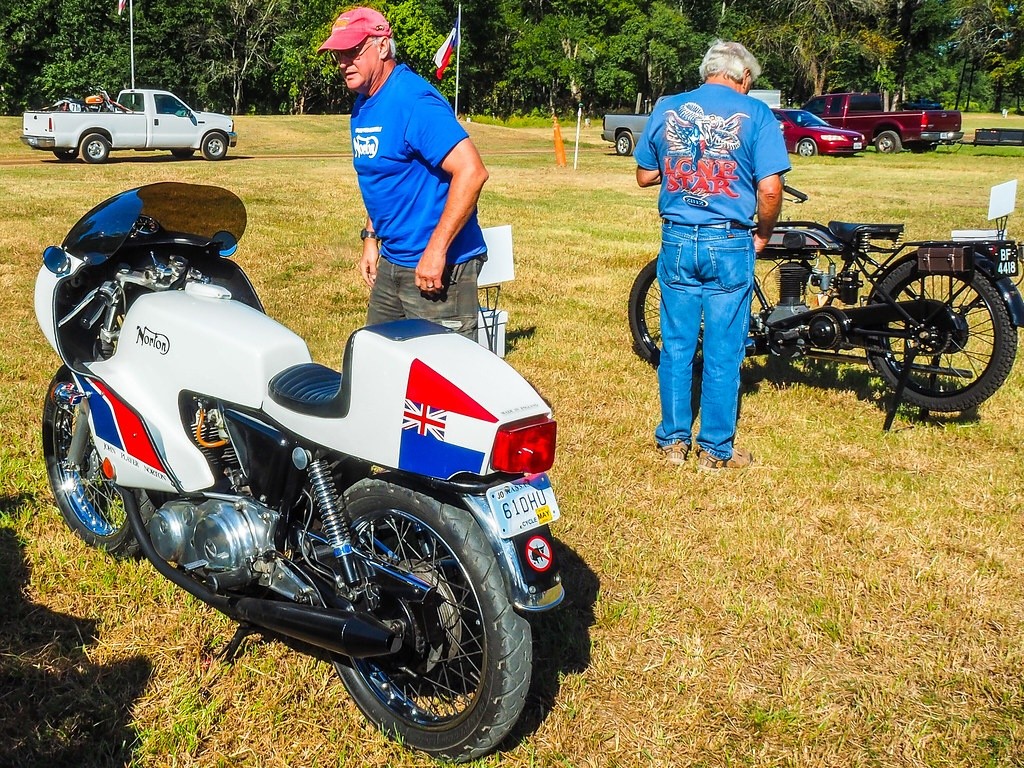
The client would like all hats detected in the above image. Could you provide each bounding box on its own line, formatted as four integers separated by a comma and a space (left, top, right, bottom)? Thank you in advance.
317, 6, 393, 57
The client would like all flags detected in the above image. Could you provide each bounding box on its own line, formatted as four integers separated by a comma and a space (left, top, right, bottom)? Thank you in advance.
432, 18, 458, 79
118, 0, 127, 15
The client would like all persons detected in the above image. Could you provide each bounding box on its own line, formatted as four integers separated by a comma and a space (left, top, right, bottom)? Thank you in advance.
317, 8, 491, 343
633, 42, 792, 472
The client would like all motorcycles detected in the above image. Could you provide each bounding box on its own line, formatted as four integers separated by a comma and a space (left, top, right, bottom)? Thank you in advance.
33, 182, 566, 763
629, 177, 1024, 432
56, 87, 117, 111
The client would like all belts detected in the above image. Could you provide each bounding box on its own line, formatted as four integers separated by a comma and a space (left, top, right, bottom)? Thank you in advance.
660, 217, 752, 231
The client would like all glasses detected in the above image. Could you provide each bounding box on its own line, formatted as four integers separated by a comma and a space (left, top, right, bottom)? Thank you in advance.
326, 37, 378, 62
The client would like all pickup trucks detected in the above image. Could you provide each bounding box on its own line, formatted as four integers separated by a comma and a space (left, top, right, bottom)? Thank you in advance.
20, 89, 237, 162
600, 96, 672, 156
799, 92, 965, 154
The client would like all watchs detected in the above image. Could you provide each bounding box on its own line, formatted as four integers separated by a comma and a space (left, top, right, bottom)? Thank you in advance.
360, 228, 375, 241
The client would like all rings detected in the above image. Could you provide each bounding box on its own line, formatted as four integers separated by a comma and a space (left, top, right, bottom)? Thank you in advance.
427, 285, 434, 288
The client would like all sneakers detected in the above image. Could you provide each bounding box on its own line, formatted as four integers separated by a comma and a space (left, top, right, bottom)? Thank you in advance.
659, 442, 689, 464
697, 446, 754, 473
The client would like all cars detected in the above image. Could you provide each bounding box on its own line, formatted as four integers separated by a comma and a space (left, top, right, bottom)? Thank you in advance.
770, 108, 868, 157
